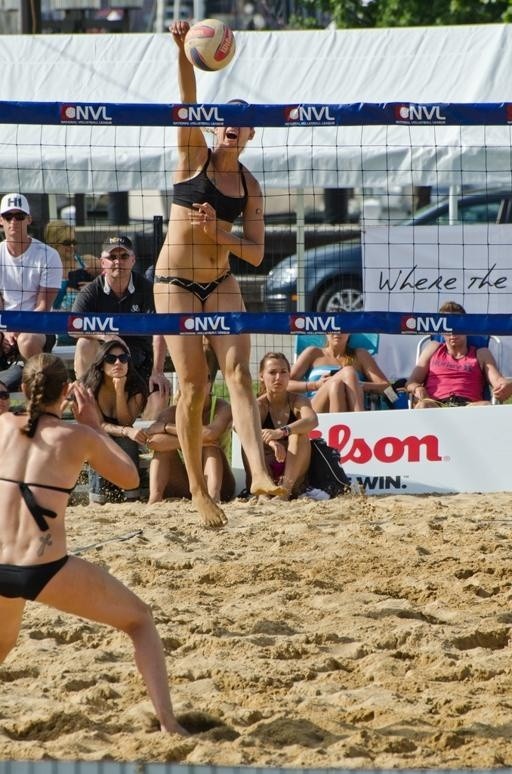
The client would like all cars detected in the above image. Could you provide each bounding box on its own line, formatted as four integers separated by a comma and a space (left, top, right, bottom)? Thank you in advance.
262, 182, 512, 314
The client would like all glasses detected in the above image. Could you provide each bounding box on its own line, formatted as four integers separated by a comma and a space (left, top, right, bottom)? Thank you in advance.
5, 212, 26, 220
104, 254, 129, 260
103, 354, 129, 364
62, 240, 78, 246
0, 391, 9, 400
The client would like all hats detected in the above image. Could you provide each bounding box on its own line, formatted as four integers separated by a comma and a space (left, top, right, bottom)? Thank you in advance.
102, 234, 132, 253
0, 193, 29, 215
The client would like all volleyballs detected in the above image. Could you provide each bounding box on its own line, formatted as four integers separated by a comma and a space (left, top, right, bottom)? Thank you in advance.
184, 18, 235, 71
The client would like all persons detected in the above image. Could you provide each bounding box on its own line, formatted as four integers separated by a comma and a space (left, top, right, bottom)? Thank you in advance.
285, 308, 389, 415
149, 17, 290, 531
1, 348, 204, 740
406, 303, 508, 409
0, 188, 355, 499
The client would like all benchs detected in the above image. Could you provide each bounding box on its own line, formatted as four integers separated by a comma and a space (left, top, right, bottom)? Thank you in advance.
1, 340, 173, 497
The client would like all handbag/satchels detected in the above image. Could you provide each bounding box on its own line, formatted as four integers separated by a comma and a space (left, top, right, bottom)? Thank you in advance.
310, 438, 350, 490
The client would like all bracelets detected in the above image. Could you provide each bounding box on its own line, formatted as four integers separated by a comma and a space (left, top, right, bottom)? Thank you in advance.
413, 383, 424, 393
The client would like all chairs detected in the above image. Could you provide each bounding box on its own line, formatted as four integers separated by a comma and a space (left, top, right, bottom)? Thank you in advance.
408, 331, 505, 409
294, 332, 380, 409
